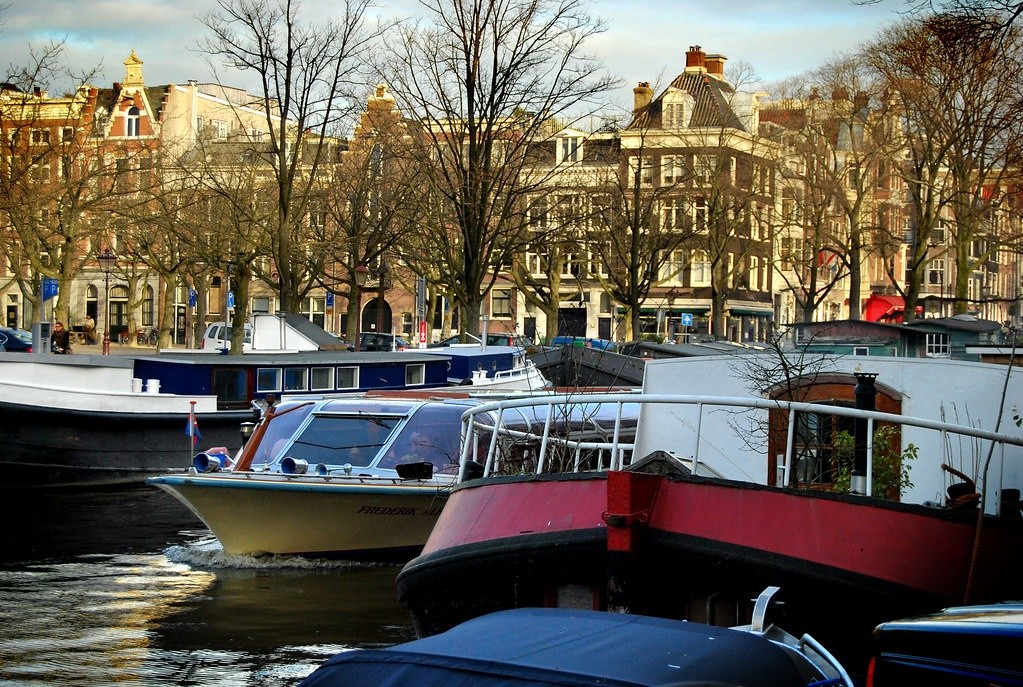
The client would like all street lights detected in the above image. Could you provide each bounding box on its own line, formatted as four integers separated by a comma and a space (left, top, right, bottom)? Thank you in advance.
352, 260, 368, 351
97, 247, 116, 355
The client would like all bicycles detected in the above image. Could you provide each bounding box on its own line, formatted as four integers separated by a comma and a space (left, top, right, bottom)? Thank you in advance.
121, 325, 159, 346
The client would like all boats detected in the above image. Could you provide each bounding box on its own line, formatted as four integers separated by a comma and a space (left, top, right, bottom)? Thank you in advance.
526, 329, 776, 387
0, 312, 552, 487
143, 387, 640, 556
394, 350, 1022, 663
297, 583, 856, 687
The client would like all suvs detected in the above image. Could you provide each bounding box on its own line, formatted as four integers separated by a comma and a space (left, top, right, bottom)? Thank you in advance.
426, 334, 538, 356
860, 600, 1022, 687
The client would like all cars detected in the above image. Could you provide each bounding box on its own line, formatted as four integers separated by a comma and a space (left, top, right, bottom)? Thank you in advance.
199, 322, 252, 351
357, 329, 411, 351
0, 325, 32, 352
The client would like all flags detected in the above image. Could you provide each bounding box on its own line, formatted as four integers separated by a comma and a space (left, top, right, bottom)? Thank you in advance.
826, 255, 842, 275
185, 414, 201, 448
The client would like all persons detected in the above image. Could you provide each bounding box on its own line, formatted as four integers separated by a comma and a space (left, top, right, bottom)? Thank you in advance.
586, 339, 592, 347
86, 316, 94, 327
51, 322, 71, 354
341, 333, 347, 339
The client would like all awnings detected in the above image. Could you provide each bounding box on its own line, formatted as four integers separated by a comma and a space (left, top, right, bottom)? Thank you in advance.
867, 295, 905, 322
